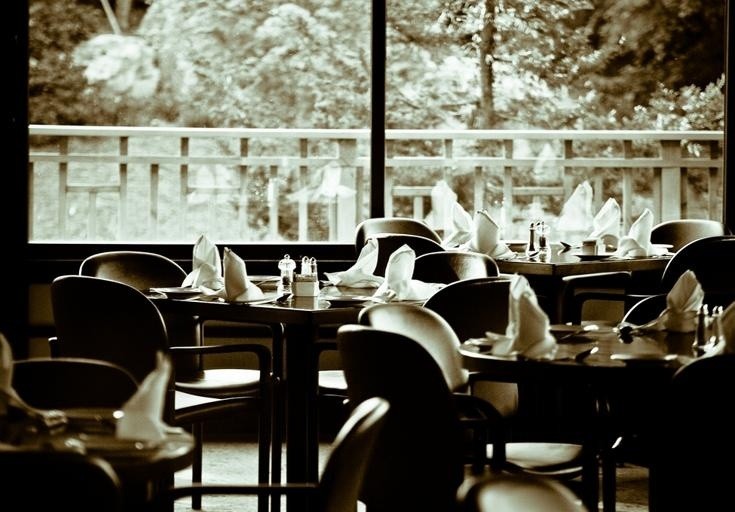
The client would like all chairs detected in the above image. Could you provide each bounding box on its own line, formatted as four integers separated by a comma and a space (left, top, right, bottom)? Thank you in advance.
0, 213, 733, 512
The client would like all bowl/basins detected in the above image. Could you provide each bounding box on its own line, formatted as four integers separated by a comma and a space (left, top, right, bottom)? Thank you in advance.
508, 242, 528, 252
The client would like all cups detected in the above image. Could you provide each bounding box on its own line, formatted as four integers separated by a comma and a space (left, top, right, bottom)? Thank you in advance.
582, 241, 598, 254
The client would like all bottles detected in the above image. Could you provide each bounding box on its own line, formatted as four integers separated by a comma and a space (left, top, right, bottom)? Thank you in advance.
529, 219, 548, 251
711, 306, 721, 345
692, 306, 708, 351
277, 251, 320, 299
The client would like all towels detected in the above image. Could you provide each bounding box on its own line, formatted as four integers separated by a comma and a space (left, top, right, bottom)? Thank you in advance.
428, 176, 471, 230
559, 179, 594, 229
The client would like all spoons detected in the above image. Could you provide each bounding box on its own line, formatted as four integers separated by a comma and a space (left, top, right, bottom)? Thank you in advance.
555, 346, 600, 362
516, 250, 541, 260
254, 294, 292, 305
558, 249, 572, 256
559, 241, 582, 249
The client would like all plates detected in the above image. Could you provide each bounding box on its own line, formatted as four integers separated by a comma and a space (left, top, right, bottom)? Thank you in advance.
153, 287, 205, 299
86, 443, 163, 461
574, 254, 611, 260
549, 324, 613, 338
470, 338, 494, 349
322, 294, 369, 307
248, 277, 282, 288
609, 352, 679, 363
